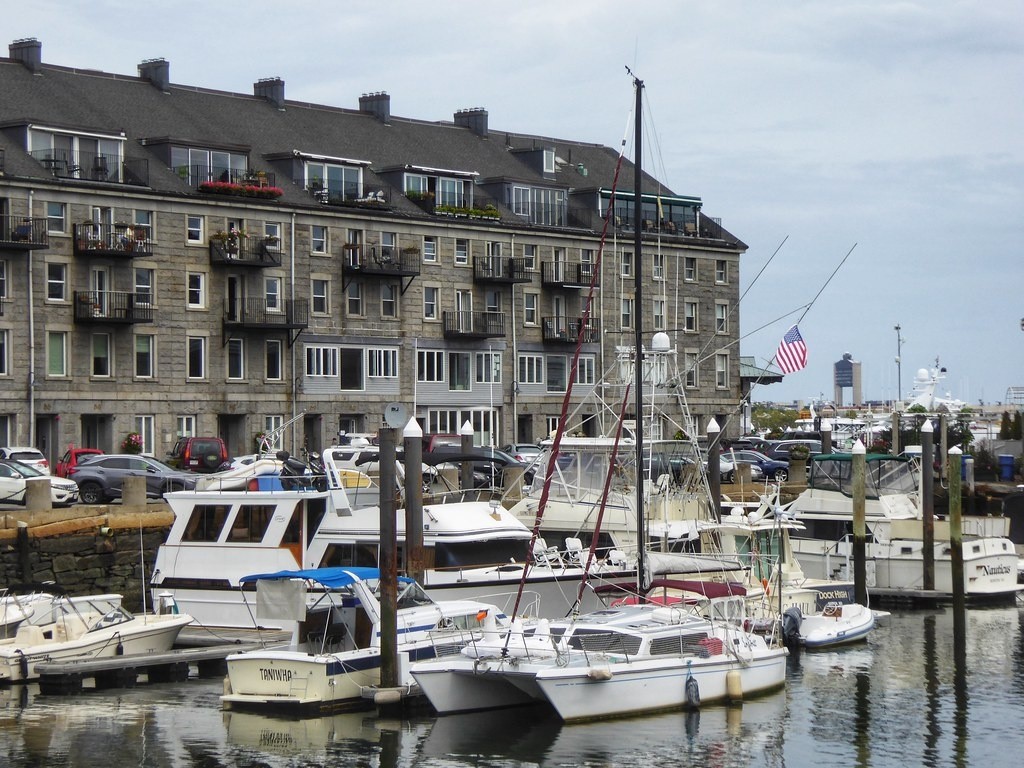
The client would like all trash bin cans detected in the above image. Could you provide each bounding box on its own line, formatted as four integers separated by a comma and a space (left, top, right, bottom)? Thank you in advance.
962, 455, 973, 481
999, 454, 1015, 481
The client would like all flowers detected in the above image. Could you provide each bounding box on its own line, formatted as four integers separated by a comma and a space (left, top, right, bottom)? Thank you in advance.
227, 228, 250, 247
254, 432, 271, 454
122, 432, 144, 454
258, 172, 265, 174
199, 182, 284, 195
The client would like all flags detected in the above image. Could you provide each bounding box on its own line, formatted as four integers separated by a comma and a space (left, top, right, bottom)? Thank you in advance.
776, 324, 807, 374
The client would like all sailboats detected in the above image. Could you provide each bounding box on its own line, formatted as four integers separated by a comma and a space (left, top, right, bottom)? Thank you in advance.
408, 64, 789, 728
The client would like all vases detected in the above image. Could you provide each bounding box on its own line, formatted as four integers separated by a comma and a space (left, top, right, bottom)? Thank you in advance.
259, 174, 262, 177
202, 190, 281, 199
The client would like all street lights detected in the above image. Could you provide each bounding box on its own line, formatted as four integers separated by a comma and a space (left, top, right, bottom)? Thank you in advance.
894, 324, 905, 399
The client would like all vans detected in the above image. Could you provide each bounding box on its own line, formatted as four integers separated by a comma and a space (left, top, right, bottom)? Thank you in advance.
419, 434, 461, 454
323, 439, 457, 493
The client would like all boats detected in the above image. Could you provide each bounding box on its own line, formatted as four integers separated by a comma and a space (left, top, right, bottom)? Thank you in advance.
1, 516, 194, 680
508, 439, 1024, 633
148, 474, 533, 632
798, 602, 874, 649
219, 567, 541, 706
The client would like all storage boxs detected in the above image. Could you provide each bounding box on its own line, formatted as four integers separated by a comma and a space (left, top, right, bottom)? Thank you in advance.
276, 451, 289, 460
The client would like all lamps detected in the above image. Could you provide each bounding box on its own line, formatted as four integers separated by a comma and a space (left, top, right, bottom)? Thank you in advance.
296, 377, 303, 390
510, 380, 521, 402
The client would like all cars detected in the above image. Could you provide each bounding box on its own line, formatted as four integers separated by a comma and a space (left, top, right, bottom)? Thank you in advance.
212, 455, 313, 475
502, 444, 549, 473
695, 451, 762, 483
615, 455, 709, 487
723, 451, 790, 482
0, 447, 194, 504
451, 462, 490, 494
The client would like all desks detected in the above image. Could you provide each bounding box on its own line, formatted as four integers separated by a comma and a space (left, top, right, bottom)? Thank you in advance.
40, 159, 69, 177
115, 308, 134, 318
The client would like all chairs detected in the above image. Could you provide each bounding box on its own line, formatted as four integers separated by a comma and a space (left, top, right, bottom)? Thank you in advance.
17, 218, 31, 242
307, 623, 348, 654
615, 216, 699, 237
232, 527, 249, 543
79, 295, 101, 316
354, 191, 386, 203
89, 234, 147, 253
480, 261, 495, 277
529, 538, 634, 574
44, 155, 110, 181
652, 473, 669, 495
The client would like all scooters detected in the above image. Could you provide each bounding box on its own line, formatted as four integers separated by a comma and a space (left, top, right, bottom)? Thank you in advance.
276, 450, 329, 493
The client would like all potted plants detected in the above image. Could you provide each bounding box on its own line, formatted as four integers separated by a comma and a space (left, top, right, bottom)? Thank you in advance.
406, 190, 501, 221
241, 169, 260, 187
313, 175, 325, 189
210, 230, 228, 242
405, 245, 419, 253
788, 444, 810, 461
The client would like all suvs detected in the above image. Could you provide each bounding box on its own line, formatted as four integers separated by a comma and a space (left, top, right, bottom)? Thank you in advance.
164, 437, 228, 473
431, 445, 538, 487
721, 430, 852, 471
